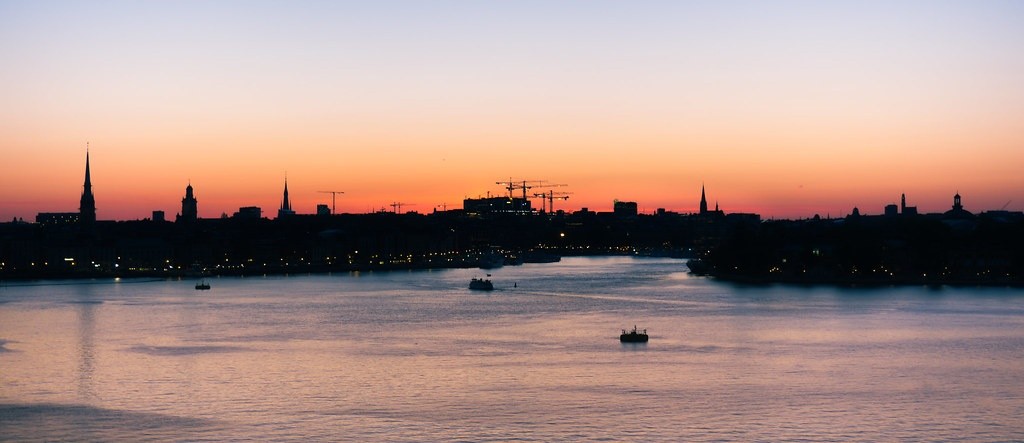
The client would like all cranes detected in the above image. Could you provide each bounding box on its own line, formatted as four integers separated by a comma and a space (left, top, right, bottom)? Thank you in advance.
497, 178, 575, 213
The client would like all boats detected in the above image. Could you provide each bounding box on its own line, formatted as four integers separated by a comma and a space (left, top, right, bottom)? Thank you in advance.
467, 275, 493, 291
194, 278, 210, 290
620, 325, 649, 343
